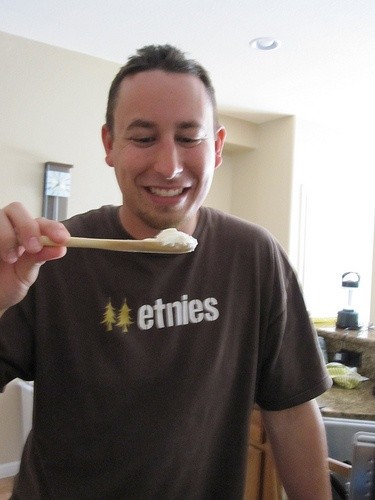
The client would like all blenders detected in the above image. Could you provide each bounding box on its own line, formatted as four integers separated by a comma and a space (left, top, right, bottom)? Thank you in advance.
336, 269, 362, 330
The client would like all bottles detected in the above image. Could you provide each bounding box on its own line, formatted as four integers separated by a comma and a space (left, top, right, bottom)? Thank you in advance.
333, 350, 342, 364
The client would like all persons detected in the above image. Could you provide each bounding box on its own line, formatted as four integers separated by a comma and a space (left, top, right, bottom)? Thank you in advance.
0, 45, 332, 499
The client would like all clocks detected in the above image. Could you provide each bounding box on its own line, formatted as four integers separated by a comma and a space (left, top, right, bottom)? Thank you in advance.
46, 171, 71, 198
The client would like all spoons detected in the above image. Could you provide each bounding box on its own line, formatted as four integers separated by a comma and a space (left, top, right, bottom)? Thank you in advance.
14, 233, 197, 256
